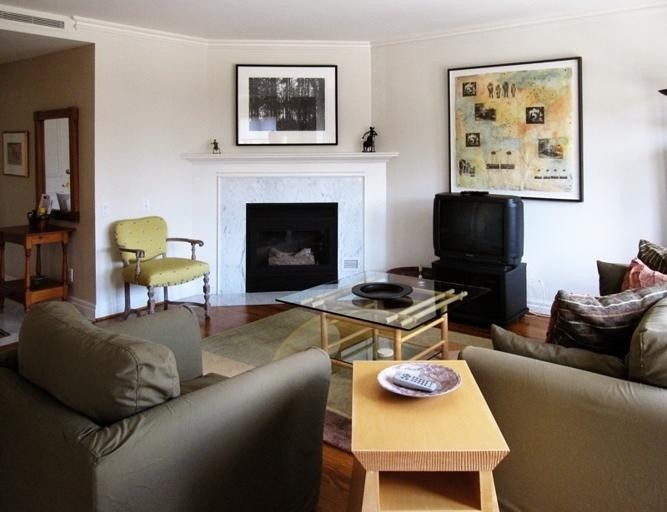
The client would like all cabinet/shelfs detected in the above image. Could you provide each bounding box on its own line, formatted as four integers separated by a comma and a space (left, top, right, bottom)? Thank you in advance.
428, 259, 529, 329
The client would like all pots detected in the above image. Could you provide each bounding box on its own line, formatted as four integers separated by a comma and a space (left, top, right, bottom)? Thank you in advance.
27, 211, 49, 231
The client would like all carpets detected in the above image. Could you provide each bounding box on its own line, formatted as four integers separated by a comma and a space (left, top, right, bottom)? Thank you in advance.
201, 306, 497, 462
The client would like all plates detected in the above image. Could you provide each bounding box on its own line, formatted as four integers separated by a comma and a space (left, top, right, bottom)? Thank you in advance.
377, 362, 462, 398
352, 296, 413, 310
351, 282, 413, 299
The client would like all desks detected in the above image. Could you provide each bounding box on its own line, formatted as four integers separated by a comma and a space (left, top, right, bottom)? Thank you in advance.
0, 223, 77, 315
350, 358, 511, 511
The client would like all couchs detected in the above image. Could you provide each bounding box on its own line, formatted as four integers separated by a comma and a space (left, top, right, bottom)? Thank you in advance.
453, 257, 666, 511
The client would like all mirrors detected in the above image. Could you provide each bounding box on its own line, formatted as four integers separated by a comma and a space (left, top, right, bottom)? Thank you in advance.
32, 106, 80, 219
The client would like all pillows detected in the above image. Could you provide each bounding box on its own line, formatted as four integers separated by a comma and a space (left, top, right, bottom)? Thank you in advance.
544, 285, 667, 360
636, 239, 667, 275
617, 257, 666, 293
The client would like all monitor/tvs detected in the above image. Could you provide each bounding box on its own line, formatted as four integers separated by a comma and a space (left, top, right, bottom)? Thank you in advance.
434, 192, 524, 271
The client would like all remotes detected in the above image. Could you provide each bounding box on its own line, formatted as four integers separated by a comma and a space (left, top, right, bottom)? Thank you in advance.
393, 372, 436, 392
461, 191, 488, 195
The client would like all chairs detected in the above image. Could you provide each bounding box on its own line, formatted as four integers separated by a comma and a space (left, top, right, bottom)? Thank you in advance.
108, 211, 212, 329
0, 294, 334, 511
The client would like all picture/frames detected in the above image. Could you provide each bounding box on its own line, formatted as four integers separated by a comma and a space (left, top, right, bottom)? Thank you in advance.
235, 64, 340, 147
447, 54, 584, 204
1, 129, 29, 179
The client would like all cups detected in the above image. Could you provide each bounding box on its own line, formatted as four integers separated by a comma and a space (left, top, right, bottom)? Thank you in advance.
32, 277, 42, 286
